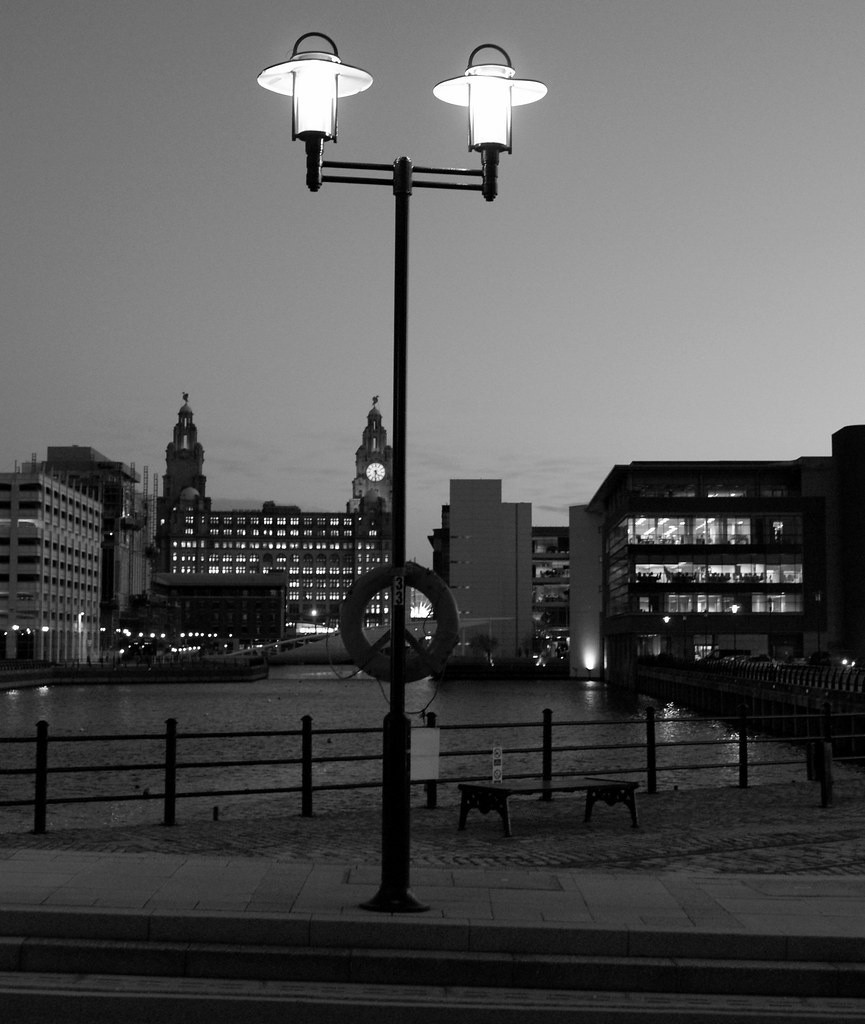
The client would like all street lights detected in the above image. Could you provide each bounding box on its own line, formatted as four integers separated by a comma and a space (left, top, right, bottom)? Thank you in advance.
256, 31, 547, 917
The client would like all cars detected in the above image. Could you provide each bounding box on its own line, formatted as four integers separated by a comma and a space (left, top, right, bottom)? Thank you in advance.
702, 648, 856, 670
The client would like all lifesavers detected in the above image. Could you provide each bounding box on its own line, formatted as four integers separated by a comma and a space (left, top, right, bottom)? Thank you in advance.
339, 561, 460, 685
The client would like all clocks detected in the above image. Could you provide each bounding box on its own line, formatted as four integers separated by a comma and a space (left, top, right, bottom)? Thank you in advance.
365, 461, 387, 482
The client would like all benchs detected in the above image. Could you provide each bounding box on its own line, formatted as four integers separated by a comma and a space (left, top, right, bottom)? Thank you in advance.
456, 776, 641, 837
